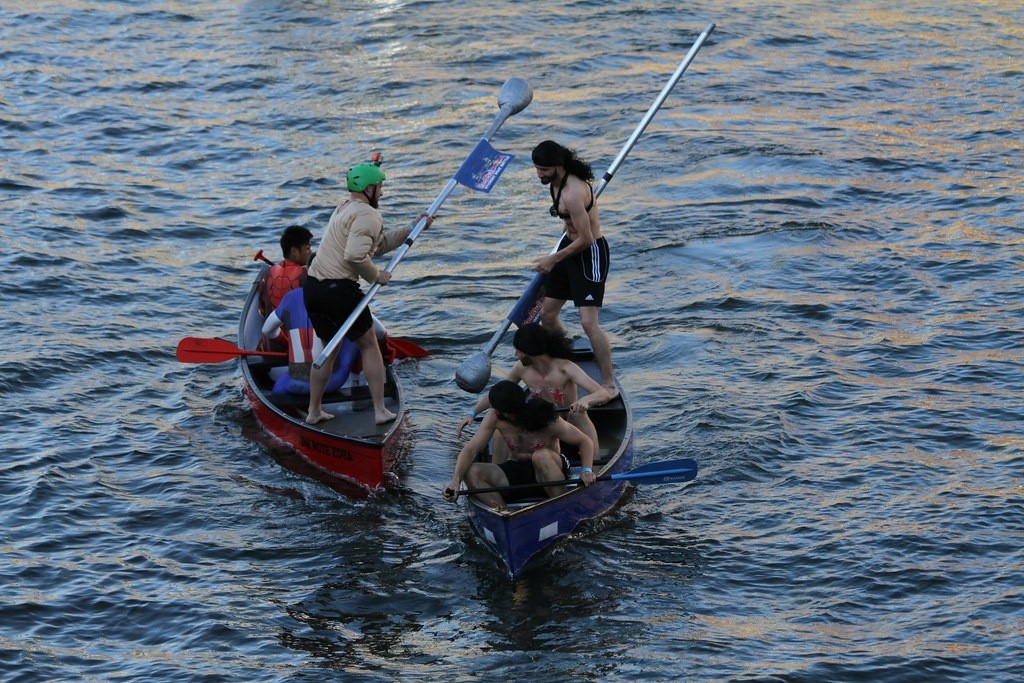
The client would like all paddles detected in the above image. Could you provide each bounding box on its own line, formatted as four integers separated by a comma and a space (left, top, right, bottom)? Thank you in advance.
177, 337, 288, 364
446, 459, 698, 496
254, 249, 432, 358
473, 406, 574, 422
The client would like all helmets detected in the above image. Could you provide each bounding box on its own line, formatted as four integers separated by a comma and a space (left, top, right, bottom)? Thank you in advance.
347, 163, 386, 192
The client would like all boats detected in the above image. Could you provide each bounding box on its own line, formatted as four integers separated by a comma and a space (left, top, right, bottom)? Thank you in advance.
238, 261, 404, 487
461, 334, 635, 577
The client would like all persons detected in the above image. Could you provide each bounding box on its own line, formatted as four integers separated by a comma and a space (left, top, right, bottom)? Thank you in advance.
302, 163, 435, 424
528, 140, 619, 406
457, 322, 611, 470
261, 226, 396, 411
441, 380, 596, 510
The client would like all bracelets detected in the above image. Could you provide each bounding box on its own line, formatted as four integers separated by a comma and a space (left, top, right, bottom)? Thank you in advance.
467, 410, 477, 417
409, 224, 415, 231
581, 468, 592, 473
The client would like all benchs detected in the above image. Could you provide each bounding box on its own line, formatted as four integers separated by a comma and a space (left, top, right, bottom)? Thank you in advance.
257, 366, 398, 407
